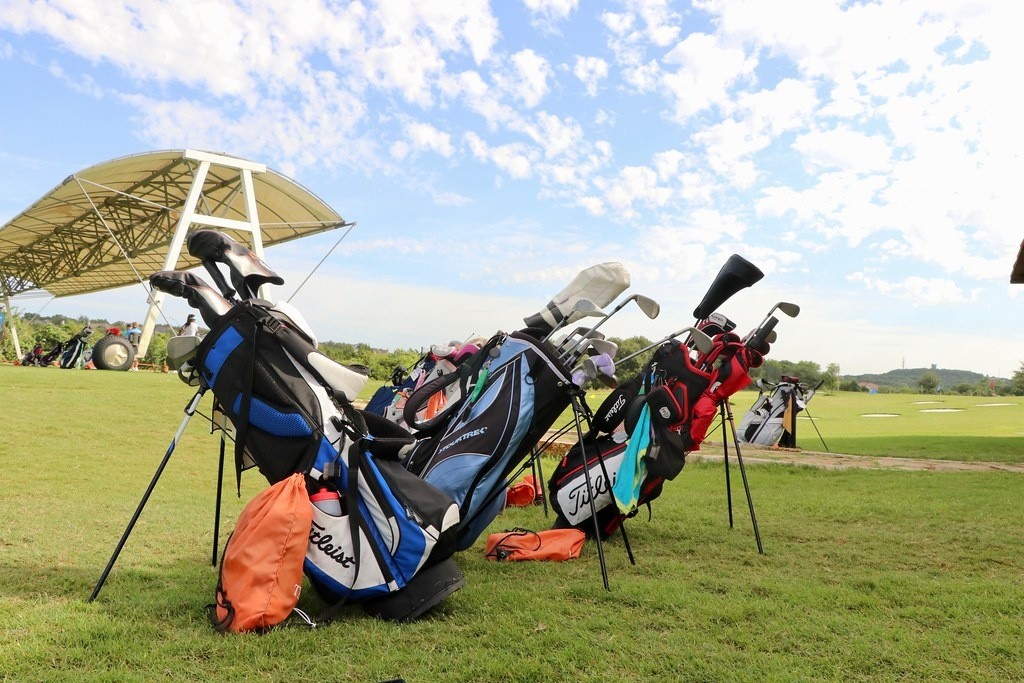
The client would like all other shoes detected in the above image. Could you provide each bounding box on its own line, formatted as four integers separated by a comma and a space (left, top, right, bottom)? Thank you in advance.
134, 367, 138, 371
129, 368, 134, 370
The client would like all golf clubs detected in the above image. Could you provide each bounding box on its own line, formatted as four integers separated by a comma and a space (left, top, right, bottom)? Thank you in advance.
743, 301, 802, 346
801, 382, 808, 391
540, 293, 715, 381
164, 334, 201, 388
168, 315, 182, 327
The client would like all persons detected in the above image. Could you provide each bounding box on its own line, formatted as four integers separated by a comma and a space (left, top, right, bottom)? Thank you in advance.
0, 304, 8, 339
182, 313, 198, 336
121, 321, 142, 371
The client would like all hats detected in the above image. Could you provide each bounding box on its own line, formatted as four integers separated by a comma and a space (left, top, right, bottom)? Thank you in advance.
190, 316, 197, 320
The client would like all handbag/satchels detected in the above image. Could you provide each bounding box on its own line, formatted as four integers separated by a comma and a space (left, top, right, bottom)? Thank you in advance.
485, 527, 585, 561
506, 473, 542, 507
205, 472, 314, 634
